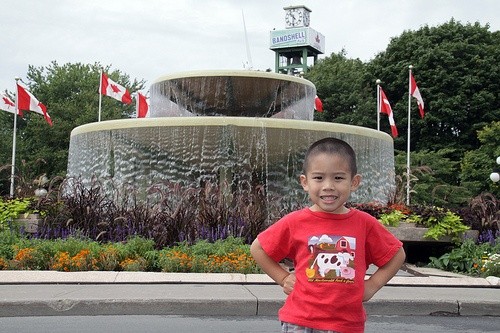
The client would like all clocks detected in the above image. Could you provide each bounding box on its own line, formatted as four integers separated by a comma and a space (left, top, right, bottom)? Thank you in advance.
283, 6, 303, 28
303, 6, 313, 27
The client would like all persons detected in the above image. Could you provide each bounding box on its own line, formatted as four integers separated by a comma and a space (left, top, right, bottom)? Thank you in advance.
249, 136, 406, 333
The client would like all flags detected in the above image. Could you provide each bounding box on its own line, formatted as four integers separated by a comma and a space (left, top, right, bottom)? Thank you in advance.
137, 93, 150, 118
410, 75, 426, 119
379, 86, 399, 138
99, 72, 133, 105
0, 90, 23, 117
16, 83, 52, 126
314, 94, 323, 112
